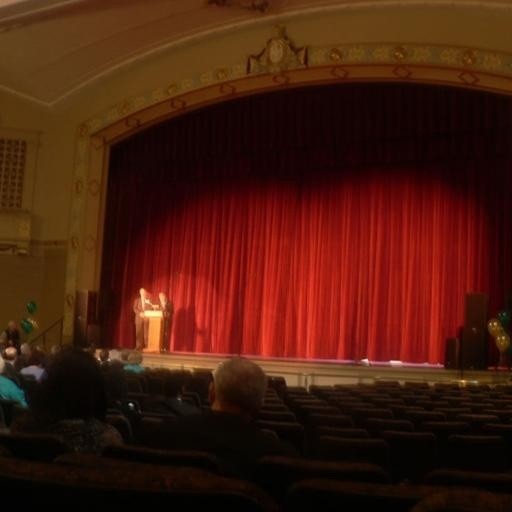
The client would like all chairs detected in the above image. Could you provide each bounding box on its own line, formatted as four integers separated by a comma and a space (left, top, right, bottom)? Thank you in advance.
2, 369, 510, 512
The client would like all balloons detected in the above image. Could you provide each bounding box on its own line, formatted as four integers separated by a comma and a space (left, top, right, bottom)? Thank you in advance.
496, 309, 511, 328
487, 318, 502, 339
495, 332, 510, 353
19, 301, 41, 334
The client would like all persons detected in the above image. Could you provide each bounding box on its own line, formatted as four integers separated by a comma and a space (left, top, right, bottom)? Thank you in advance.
133, 288, 152, 352
157, 292, 175, 350
